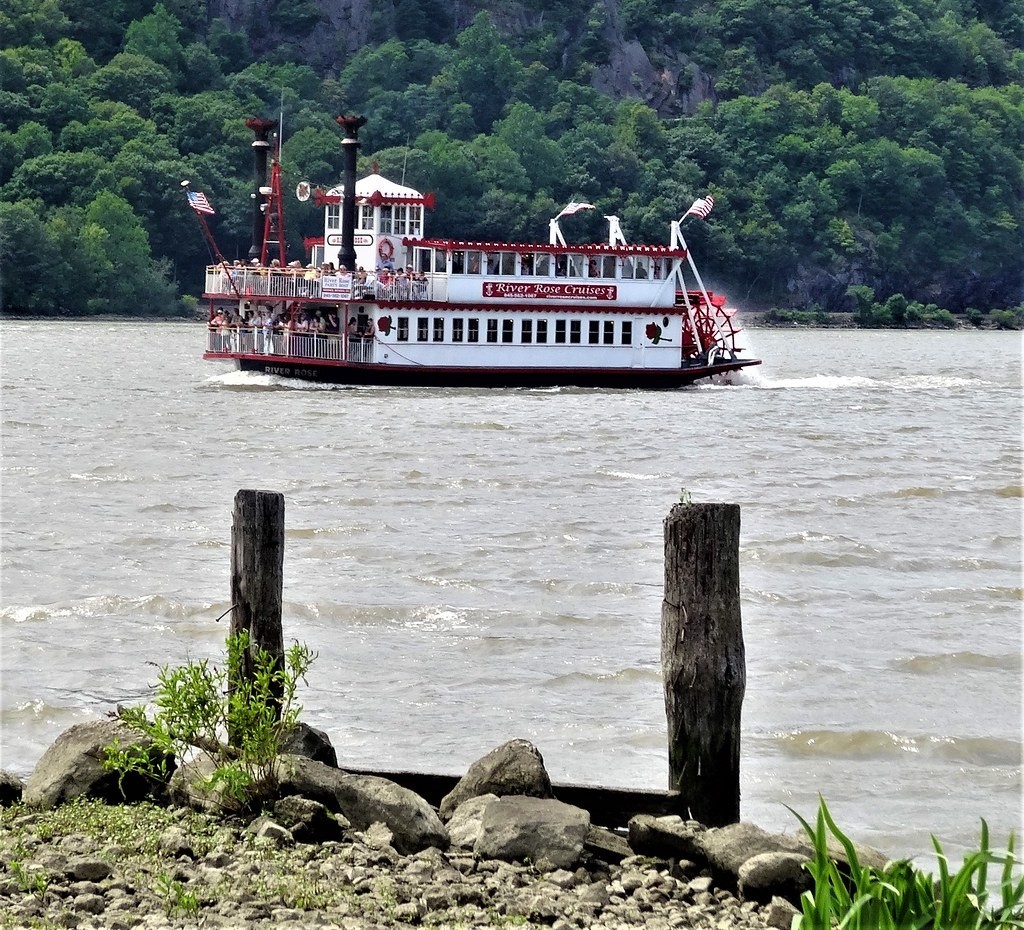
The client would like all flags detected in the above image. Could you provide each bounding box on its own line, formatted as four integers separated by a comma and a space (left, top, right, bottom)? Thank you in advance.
187, 192, 214, 214
689, 195, 714, 218
562, 202, 595, 214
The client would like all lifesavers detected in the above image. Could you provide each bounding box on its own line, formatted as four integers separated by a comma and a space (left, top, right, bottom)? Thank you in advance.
379, 239, 394, 260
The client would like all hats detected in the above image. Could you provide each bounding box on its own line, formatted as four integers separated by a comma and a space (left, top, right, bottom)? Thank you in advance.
407, 264, 412, 268
397, 268, 404, 273
218, 310, 222, 312
251, 258, 259, 263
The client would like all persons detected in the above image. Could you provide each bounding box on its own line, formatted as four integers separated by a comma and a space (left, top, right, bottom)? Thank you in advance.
589, 259, 600, 278
374, 252, 429, 300
632, 262, 647, 279
211, 308, 374, 360
262, 312, 275, 356
570, 259, 576, 277
487, 259, 495, 274
297, 264, 316, 287
218, 258, 365, 297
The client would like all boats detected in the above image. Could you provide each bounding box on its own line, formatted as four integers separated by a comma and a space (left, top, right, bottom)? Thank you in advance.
179, 80, 762, 388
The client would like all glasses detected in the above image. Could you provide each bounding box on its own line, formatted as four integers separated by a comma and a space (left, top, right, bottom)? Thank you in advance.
218, 313, 222, 314
